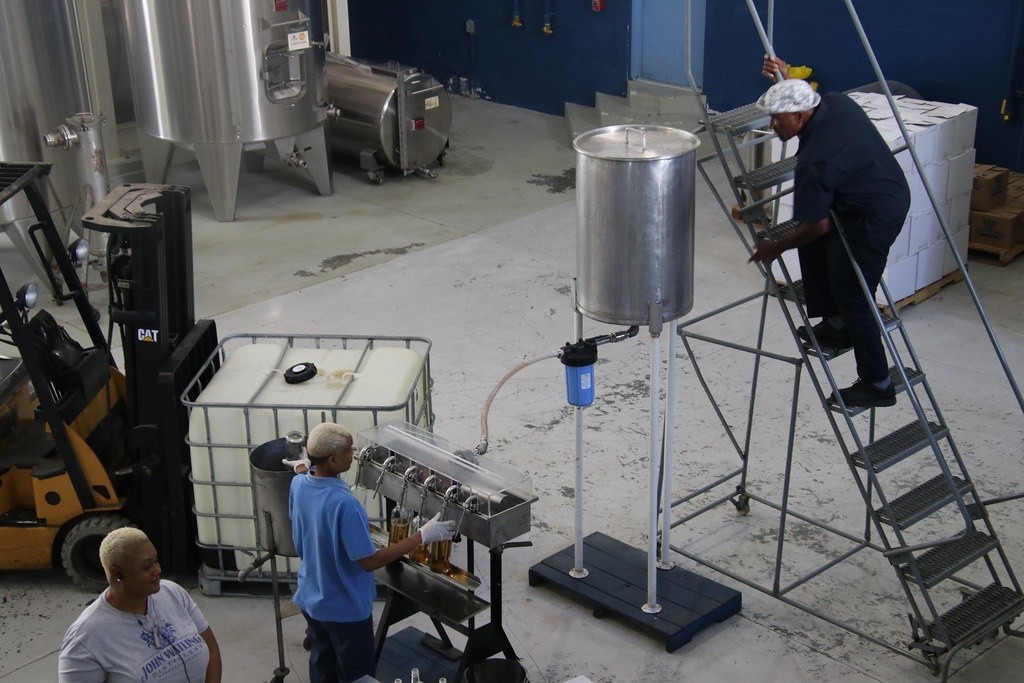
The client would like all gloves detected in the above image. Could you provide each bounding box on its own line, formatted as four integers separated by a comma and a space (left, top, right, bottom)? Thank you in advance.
417, 512, 456, 545
281, 446, 311, 473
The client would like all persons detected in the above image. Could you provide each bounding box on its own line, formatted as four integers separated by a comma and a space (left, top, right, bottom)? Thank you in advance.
58, 527, 222, 683
746, 55, 911, 408
288, 422, 456, 683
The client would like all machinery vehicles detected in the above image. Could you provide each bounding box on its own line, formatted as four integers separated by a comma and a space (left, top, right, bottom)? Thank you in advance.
0, 162, 236, 593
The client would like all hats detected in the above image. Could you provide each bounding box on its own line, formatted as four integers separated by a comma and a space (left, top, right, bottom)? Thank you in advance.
755, 78, 821, 114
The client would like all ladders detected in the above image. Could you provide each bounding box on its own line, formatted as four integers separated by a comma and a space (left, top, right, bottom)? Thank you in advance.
651, 0, 1023, 683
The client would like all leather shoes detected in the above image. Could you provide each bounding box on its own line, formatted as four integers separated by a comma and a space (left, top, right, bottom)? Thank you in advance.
831, 379, 896, 408
796, 321, 854, 349
